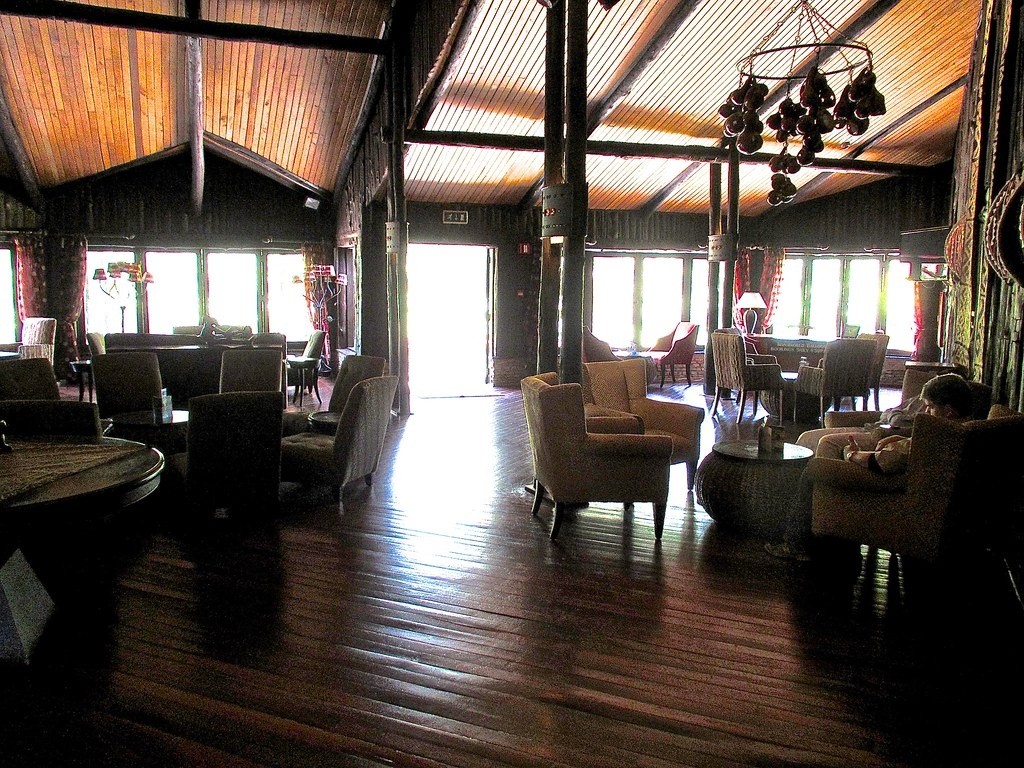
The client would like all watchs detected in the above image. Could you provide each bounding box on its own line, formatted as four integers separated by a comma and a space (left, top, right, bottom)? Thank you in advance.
846, 451, 855, 462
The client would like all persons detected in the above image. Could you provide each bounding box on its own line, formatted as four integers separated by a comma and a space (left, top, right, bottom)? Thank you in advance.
762, 371, 972, 564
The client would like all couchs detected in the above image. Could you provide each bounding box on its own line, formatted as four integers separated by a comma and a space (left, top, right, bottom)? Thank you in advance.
519, 372, 675, 541
824, 369, 995, 428
646, 322, 700, 388
794, 404, 1024, 601
519, 371, 646, 434
582, 357, 704, 489
0, 401, 103, 445
709, 327, 891, 427
583, 324, 623, 362
91, 351, 163, 419
282, 329, 326, 395
218, 350, 284, 393
281, 376, 399, 502
86, 333, 107, 357
170, 392, 285, 529
282, 355, 387, 440
0, 316, 58, 368
0, 358, 61, 400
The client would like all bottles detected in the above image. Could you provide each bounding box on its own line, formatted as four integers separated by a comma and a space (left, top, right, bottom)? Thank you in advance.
798, 357, 809, 376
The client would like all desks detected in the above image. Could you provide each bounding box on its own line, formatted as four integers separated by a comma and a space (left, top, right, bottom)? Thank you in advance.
1, 435, 165, 668
694, 440, 814, 535
306, 410, 343, 437
903, 360, 962, 378
70, 359, 94, 402
615, 352, 659, 387
759, 370, 834, 422
284, 356, 323, 408
99, 409, 190, 458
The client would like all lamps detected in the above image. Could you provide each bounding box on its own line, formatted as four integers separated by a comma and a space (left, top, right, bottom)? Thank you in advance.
92, 261, 154, 333
734, 292, 767, 335
293, 264, 349, 375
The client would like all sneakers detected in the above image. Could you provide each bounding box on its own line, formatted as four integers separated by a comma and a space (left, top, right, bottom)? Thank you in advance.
764, 543, 810, 560
